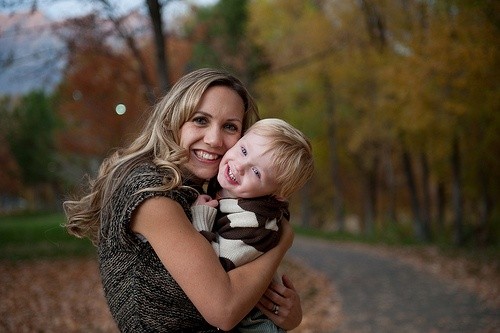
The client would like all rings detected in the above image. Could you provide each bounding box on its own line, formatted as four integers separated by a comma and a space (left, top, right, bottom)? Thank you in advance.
274, 306, 279, 315
272, 304, 276, 313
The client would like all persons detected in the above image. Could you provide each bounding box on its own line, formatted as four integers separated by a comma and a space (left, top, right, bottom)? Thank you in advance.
189, 119, 315, 333
60, 68, 303, 333
